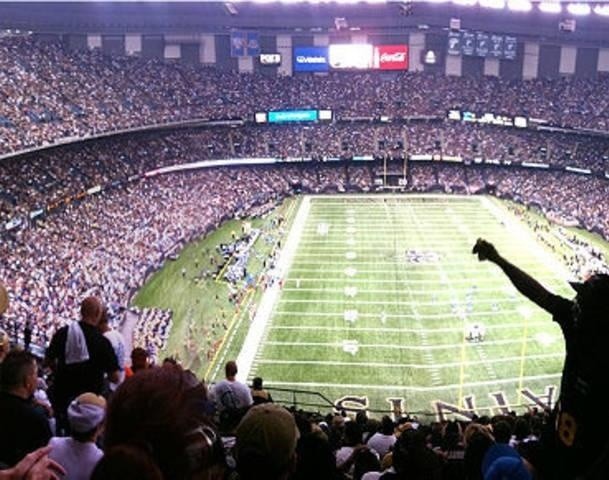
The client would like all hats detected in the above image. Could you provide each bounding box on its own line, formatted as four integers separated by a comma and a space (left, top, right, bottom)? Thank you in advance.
66, 393, 108, 432
236, 403, 300, 469
571, 273, 609, 301
392, 429, 427, 471
480, 443, 532, 479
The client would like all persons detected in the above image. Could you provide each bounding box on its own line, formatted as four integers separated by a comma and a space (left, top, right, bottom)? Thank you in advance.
1, 31, 608, 240
1, 216, 609, 480
